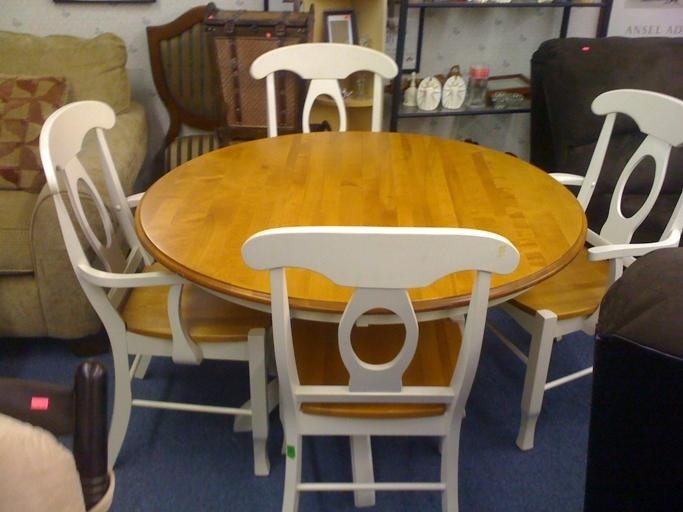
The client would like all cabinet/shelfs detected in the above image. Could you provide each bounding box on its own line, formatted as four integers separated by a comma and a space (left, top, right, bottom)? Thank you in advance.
392, 1, 613, 131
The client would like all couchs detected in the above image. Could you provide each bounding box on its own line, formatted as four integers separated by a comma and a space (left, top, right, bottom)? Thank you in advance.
527, 35, 683, 272
581, 248, 682, 511
0, 29, 150, 359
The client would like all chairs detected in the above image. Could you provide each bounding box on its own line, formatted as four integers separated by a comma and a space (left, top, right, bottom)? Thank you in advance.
249, 42, 399, 137
1, 359, 111, 512
482, 87, 683, 451
38, 99, 271, 477
241, 225, 521, 512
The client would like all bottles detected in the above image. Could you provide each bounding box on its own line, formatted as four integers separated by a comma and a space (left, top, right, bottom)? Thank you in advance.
464, 62, 492, 111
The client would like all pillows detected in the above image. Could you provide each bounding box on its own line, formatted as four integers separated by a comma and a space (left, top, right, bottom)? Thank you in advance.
0, 76, 71, 194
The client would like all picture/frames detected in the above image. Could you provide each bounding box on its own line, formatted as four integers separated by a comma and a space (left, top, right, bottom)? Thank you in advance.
322, 9, 358, 44
476, 73, 531, 94
